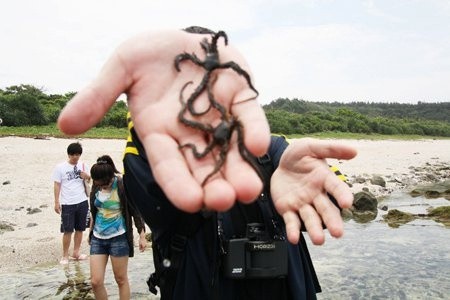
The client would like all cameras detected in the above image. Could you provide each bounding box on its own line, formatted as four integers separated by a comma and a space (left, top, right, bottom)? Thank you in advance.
226, 222, 290, 278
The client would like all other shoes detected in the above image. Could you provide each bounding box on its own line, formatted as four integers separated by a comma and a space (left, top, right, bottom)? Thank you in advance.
69, 255, 87, 260
60, 254, 70, 264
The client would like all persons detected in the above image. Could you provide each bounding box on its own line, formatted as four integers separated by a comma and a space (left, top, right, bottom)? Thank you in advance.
86, 155, 147, 300
56, 25, 358, 299
51, 141, 93, 265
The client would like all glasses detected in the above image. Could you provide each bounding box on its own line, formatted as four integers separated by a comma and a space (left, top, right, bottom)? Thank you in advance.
94, 181, 109, 188
